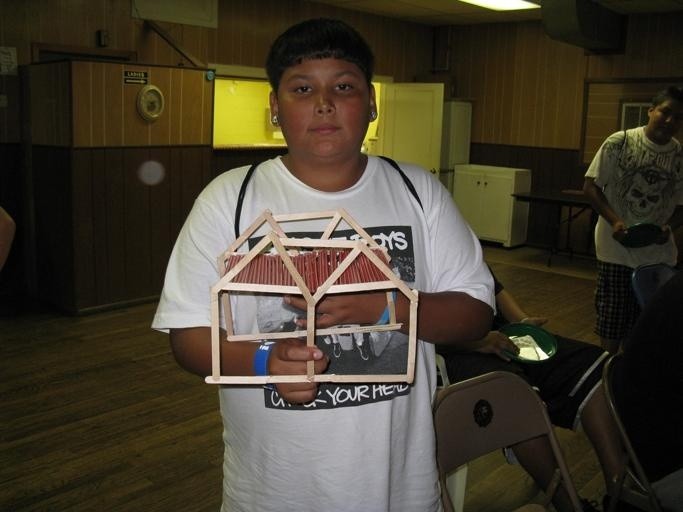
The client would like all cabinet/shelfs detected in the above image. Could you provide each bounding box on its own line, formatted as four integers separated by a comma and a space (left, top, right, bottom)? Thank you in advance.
440, 101, 472, 195
452, 164, 532, 248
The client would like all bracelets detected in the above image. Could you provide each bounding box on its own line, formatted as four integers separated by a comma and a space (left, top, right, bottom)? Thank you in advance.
374, 291, 397, 326
254, 340, 274, 392
520, 317, 528, 323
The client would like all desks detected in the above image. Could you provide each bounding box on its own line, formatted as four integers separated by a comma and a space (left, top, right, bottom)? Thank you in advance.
511, 191, 598, 267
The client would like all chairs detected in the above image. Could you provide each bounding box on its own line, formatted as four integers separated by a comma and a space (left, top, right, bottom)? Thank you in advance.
434, 355, 469, 509
631, 262, 676, 308
601, 351, 665, 510
434, 370, 583, 512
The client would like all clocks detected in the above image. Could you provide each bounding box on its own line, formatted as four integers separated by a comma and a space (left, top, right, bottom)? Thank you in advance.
137, 85, 165, 122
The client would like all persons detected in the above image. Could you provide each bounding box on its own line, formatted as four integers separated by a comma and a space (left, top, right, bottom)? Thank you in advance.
433, 260, 663, 512
148, 15, 496, 512
578, 84, 682, 356
0, 204, 16, 276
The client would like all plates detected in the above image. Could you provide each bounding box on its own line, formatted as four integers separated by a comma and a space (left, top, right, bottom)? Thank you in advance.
496, 323, 556, 364
